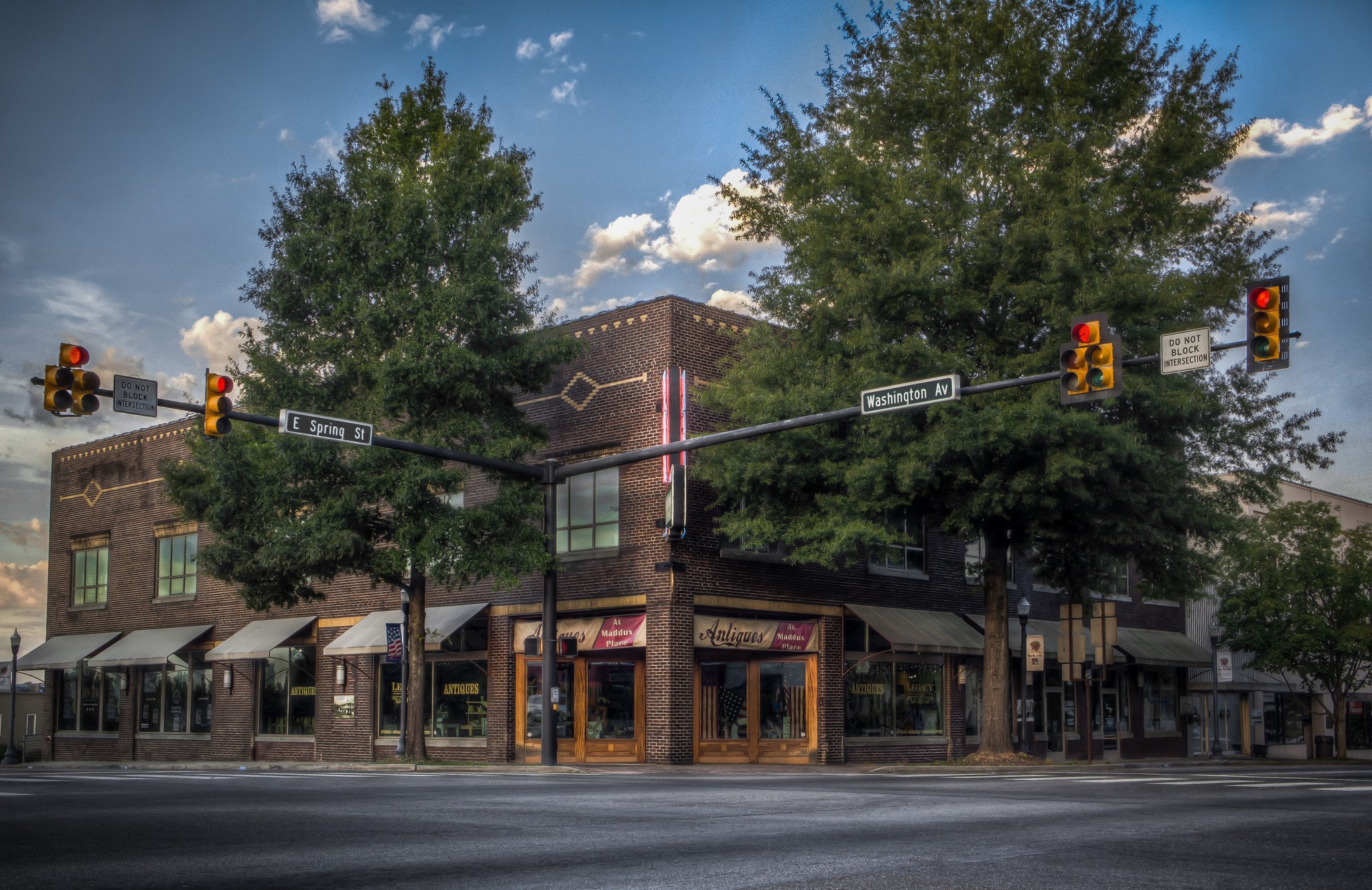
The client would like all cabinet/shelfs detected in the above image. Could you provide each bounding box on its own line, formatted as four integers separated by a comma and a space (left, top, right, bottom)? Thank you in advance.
461, 701, 487, 737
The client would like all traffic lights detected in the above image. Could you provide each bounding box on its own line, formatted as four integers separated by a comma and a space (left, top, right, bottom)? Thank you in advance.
558, 636, 578, 658
59, 342, 90, 369
43, 364, 75, 412
70, 368, 101, 416
1060, 311, 1123, 405
524, 637, 542, 657
1245, 275, 1290, 373
202, 372, 234, 438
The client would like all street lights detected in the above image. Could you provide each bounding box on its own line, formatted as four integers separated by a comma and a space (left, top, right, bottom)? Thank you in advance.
1014, 596, 1034, 756
394, 584, 412, 759
1208, 621, 1225, 761
1, 628, 22, 766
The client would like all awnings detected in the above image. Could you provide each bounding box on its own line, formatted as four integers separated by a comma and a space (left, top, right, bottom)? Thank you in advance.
11, 631, 124, 671
88, 624, 215, 668
964, 614, 1126, 663
1116, 626, 1213, 668
842, 603, 987, 656
204, 615, 318, 661
323, 603, 490, 655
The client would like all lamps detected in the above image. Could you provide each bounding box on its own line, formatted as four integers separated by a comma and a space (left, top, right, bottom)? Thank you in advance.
224, 663, 231, 696
336, 658, 345, 693
299, 648, 308, 657
120, 667, 127, 697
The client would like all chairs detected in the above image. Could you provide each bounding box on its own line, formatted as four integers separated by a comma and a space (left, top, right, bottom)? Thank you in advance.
588, 722, 603, 739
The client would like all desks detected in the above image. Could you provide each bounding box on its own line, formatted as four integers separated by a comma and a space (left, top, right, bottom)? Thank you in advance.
430, 724, 461, 737
